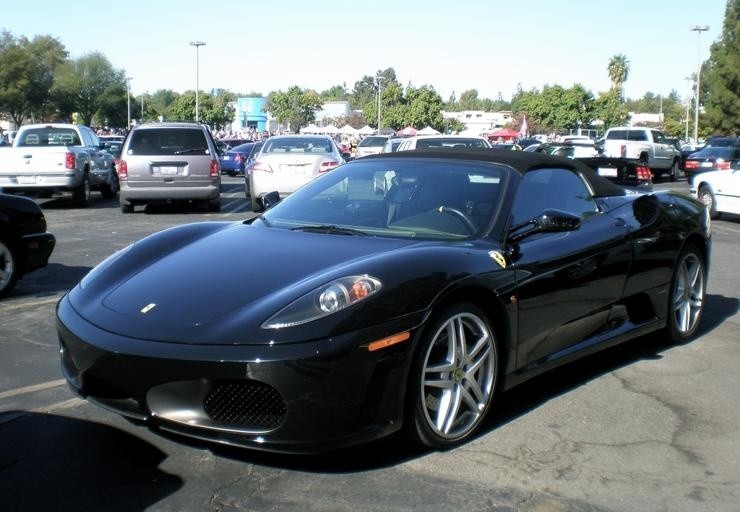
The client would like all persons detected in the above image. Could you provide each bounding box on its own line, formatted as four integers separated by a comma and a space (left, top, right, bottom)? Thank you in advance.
211, 128, 352, 160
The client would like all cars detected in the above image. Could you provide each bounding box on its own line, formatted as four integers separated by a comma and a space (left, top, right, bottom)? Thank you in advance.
594, 127, 682, 181
0, 194, 55, 294
341, 130, 603, 162
684, 136, 740, 186
113, 122, 225, 212
688, 157, 739, 220
247, 136, 349, 210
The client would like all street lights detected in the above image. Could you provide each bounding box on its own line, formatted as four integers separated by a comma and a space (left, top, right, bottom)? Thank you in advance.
689, 24, 710, 143
188, 42, 206, 123
374, 76, 387, 136
124, 77, 134, 129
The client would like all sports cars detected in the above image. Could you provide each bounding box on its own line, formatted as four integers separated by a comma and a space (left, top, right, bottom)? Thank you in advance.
52, 142, 714, 465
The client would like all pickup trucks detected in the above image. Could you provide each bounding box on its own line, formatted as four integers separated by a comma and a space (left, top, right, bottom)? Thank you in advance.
0, 122, 121, 205
